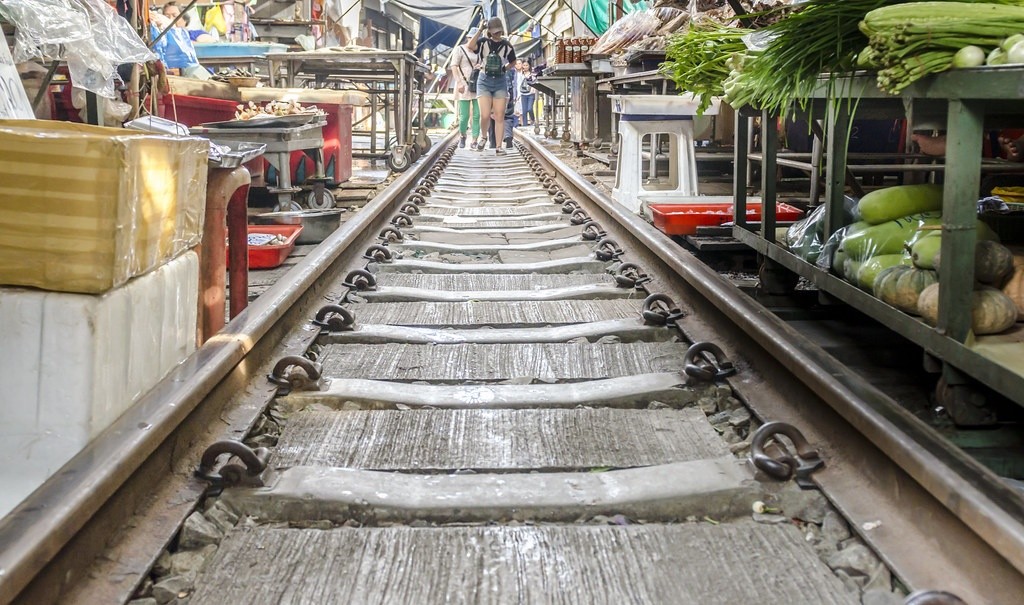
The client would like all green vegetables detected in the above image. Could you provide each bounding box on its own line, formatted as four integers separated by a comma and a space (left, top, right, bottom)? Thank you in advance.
659, 0, 910, 177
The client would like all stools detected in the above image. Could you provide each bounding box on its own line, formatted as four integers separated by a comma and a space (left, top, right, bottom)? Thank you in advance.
189, 163, 252, 350
610, 118, 697, 213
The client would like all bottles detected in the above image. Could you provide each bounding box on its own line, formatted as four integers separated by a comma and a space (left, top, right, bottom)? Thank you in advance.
555, 34, 599, 64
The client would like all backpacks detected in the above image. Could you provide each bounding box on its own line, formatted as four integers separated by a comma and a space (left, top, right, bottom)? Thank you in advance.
485, 40, 504, 77
459, 45, 479, 92
520, 74, 532, 93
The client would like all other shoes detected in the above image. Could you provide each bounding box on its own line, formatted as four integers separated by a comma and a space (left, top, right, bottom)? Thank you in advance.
471, 139, 477, 147
507, 139, 512, 148
489, 144, 495, 148
459, 136, 466, 147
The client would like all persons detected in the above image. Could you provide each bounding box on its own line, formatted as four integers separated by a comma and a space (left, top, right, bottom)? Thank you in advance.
162, 1, 217, 77
450, 17, 539, 156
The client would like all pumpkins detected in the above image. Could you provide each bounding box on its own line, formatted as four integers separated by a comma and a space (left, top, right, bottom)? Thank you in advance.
874, 240, 1024, 336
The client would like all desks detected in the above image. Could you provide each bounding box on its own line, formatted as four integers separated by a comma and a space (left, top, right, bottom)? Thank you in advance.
266, 49, 432, 172
196, 55, 270, 86
594, 63, 675, 180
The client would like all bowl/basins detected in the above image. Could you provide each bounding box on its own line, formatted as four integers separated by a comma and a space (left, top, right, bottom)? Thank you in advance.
224, 76, 261, 88
254, 207, 347, 244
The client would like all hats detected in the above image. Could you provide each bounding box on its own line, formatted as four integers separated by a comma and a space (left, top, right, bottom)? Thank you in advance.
488, 17, 503, 34
466, 27, 483, 38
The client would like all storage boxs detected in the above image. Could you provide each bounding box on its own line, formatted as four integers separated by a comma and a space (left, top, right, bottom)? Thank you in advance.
634, 193, 806, 237
0, 117, 210, 294
605, 93, 725, 116
1, 246, 200, 478
144, 94, 238, 130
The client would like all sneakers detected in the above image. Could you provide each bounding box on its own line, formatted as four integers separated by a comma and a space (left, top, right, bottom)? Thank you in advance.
478, 138, 487, 149
496, 148, 507, 155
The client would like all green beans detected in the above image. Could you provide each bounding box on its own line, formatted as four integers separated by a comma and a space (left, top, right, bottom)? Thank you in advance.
869, 12, 1024, 95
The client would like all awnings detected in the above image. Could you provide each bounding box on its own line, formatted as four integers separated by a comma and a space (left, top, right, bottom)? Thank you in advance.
1, 0, 649, 124
